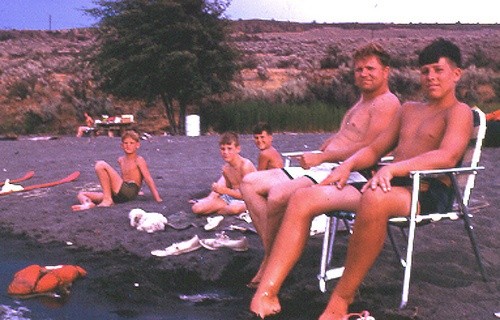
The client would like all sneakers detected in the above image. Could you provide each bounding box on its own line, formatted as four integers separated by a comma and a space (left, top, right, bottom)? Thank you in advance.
151, 235, 201, 256
199, 231, 248, 251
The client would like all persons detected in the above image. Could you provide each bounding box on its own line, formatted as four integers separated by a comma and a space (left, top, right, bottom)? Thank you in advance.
71, 130, 163, 212
251, 38, 473, 320
192, 131, 258, 216
253, 122, 283, 170
239, 46, 401, 289
76, 112, 94, 139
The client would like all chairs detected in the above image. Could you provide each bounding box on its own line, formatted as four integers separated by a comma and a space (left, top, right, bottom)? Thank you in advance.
319, 105, 490, 309
